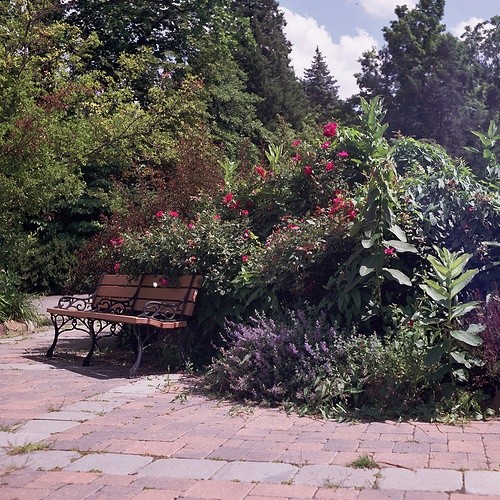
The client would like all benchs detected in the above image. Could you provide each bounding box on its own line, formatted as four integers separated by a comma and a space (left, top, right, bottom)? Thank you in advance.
44, 270, 202, 377
